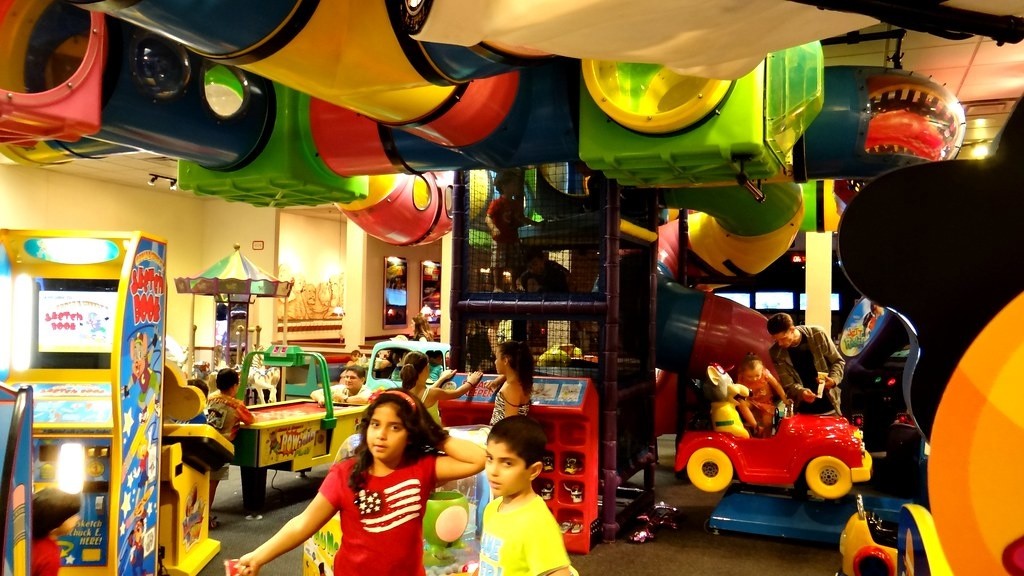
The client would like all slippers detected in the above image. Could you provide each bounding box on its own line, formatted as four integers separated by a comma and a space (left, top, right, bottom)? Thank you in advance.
560, 521, 573, 533
571, 520, 583, 533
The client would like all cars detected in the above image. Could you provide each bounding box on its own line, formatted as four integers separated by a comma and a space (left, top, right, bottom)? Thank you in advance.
672, 401, 875, 501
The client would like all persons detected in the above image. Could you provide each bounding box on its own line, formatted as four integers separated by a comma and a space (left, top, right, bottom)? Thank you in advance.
736, 351, 794, 438
484, 170, 547, 293
346, 350, 369, 366
391, 350, 408, 381
400, 351, 483, 427
521, 249, 577, 296
237, 388, 487, 576
410, 313, 434, 342
310, 365, 373, 408
173, 378, 209, 424
30, 487, 81, 576
425, 350, 444, 384
483, 340, 535, 426
479, 414, 580, 576
206, 368, 255, 529
767, 313, 846, 417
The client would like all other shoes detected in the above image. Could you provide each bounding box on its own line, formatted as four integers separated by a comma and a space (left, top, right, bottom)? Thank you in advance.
209, 516, 218, 530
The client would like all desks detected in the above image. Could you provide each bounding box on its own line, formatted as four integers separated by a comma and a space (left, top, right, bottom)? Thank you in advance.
227, 393, 370, 527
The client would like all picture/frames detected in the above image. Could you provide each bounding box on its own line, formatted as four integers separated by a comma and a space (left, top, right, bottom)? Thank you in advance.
419, 259, 441, 327
381, 255, 408, 329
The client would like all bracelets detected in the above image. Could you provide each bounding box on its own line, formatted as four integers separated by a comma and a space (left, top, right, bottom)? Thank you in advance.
344, 397, 348, 402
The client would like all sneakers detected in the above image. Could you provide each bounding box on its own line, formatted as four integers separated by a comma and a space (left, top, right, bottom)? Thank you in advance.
540, 482, 554, 500
564, 482, 583, 502
543, 456, 553, 471
565, 457, 583, 475
